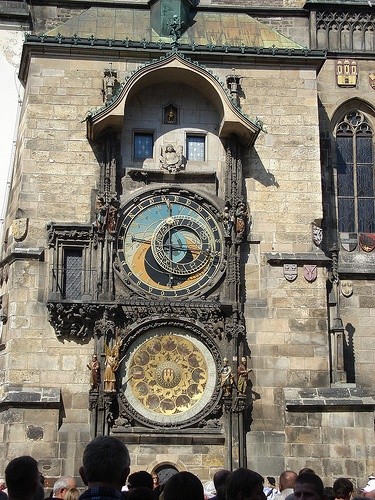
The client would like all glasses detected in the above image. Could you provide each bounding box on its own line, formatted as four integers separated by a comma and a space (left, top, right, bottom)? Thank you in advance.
38, 475, 45, 484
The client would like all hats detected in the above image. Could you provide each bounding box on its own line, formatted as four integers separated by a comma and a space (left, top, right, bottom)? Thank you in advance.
362, 479, 375, 493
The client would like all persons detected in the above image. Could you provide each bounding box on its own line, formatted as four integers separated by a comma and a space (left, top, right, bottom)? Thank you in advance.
159, 144, 183, 172
218, 355, 233, 397
237, 356, 253, 396
0, 435, 375, 500
104, 351, 120, 394
87, 353, 101, 390
